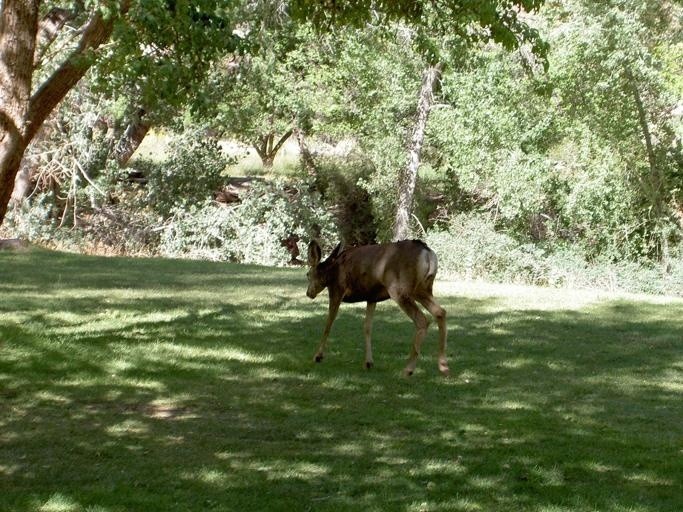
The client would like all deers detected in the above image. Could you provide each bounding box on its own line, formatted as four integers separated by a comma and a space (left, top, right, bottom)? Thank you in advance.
305, 238, 449, 380
